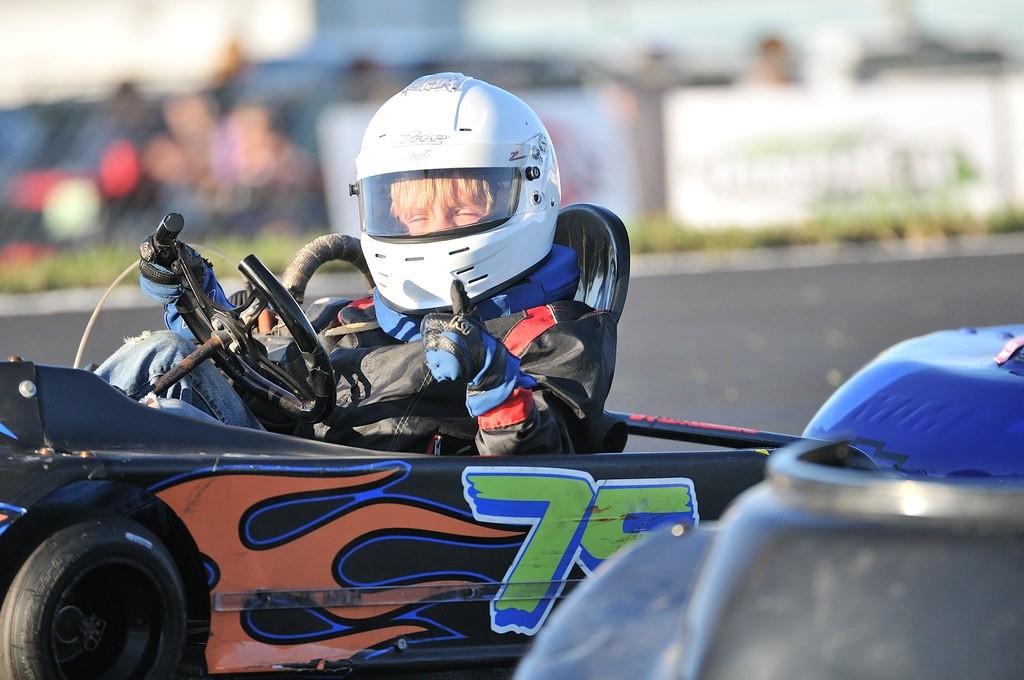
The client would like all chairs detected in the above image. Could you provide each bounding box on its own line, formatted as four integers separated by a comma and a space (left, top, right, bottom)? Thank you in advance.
553, 204, 630, 454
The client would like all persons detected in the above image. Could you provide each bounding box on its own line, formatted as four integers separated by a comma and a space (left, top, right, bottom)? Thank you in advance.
85, 72, 616, 455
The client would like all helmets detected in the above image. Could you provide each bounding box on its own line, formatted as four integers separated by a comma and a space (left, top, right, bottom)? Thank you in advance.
352, 71, 564, 312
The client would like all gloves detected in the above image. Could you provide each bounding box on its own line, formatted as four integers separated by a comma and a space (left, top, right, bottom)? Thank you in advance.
419, 279, 523, 422
138, 239, 243, 346
